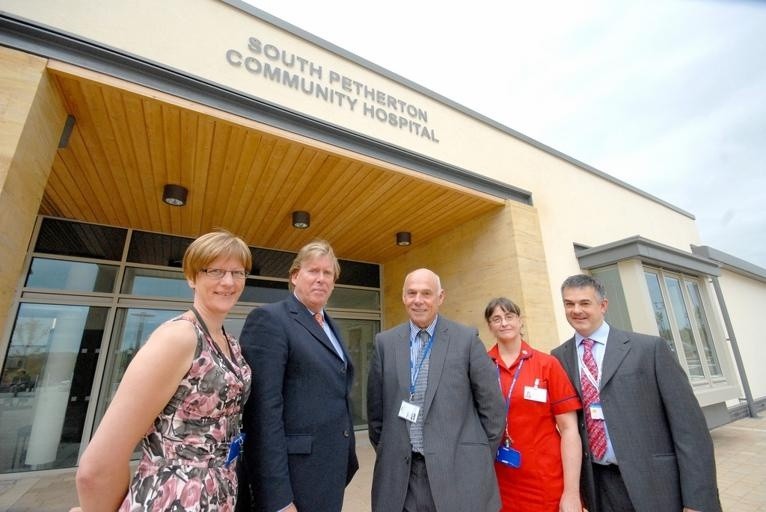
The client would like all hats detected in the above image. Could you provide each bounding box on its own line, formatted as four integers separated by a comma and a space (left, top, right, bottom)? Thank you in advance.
16, 369, 26, 375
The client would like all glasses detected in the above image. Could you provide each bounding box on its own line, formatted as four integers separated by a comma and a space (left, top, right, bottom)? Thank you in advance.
200, 267, 249, 280
488, 313, 518, 325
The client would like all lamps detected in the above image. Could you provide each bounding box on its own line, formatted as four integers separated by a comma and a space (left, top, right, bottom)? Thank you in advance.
292, 211, 309, 228
162, 184, 185, 206
395, 230, 412, 248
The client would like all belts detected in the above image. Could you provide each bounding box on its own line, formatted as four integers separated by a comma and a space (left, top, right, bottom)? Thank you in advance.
595, 464, 619, 475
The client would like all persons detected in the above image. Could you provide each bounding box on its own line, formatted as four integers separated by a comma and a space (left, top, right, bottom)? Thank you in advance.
241, 242, 359, 512
367, 268, 506, 512
550, 275, 723, 512
71, 231, 251, 512
485, 299, 583, 512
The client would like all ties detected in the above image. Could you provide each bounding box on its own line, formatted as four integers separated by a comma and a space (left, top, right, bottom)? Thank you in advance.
580, 340, 608, 460
315, 313, 325, 326
409, 331, 433, 457
